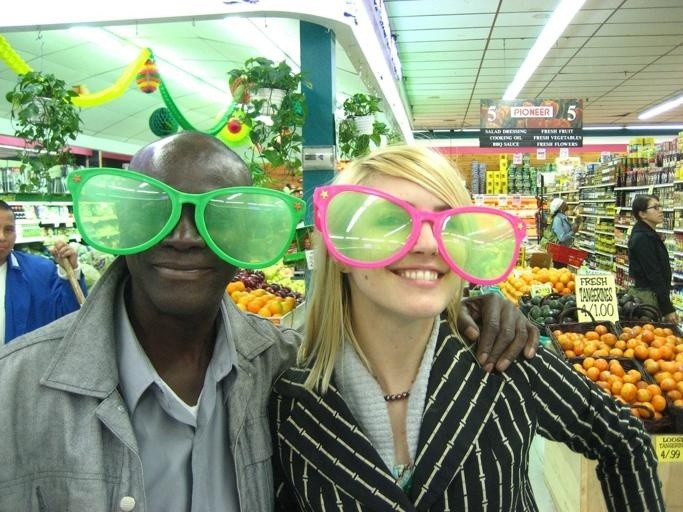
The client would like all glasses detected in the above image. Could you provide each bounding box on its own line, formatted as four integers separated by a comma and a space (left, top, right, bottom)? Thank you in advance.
310, 174, 531, 291
61, 163, 307, 278
644, 202, 662, 212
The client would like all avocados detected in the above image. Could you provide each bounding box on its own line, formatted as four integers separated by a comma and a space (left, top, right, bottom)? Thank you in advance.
529, 293, 655, 326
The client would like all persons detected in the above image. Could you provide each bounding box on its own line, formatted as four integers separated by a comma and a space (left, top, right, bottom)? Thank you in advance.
0, 133, 540, 512
269, 144, 665, 512
0, 200, 87, 344
628, 195, 680, 325
551, 198, 579, 269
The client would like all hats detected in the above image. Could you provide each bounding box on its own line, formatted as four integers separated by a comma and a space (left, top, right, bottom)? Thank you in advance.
549, 197, 564, 214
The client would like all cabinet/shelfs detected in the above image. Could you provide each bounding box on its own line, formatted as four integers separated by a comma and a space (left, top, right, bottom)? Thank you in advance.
527, 432, 683, 512
472, 178, 683, 313
0, 134, 136, 288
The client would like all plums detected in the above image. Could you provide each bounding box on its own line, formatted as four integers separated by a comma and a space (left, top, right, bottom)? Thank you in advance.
231, 269, 303, 304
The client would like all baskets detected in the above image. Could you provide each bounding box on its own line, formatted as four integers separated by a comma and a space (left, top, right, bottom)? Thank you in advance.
633, 353, 683, 433
544, 314, 628, 362
558, 353, 673, 434
614, 316, 683, 361
519, 292, 564, 310
525, 298, 578, 336
607, 290, 664, 322
544, 239, 591, 268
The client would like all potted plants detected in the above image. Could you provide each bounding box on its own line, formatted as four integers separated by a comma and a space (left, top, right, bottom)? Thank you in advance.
228, 57, 313, 187
337, 92, 390, 161
6, 70, 85, 196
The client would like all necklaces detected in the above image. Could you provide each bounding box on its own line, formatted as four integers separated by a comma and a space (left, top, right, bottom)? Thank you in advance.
384, 391, 411, 401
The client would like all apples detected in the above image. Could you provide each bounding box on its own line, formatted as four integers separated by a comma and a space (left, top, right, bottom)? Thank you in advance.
228, 120, 241, 133
481, 100, 582, 128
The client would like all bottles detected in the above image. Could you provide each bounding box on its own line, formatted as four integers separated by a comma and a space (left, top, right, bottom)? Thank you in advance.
578, 137, 682, 299
0, 166, 68, 196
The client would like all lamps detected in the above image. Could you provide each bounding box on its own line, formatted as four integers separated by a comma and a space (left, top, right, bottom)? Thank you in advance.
636, 90, 683, 121
